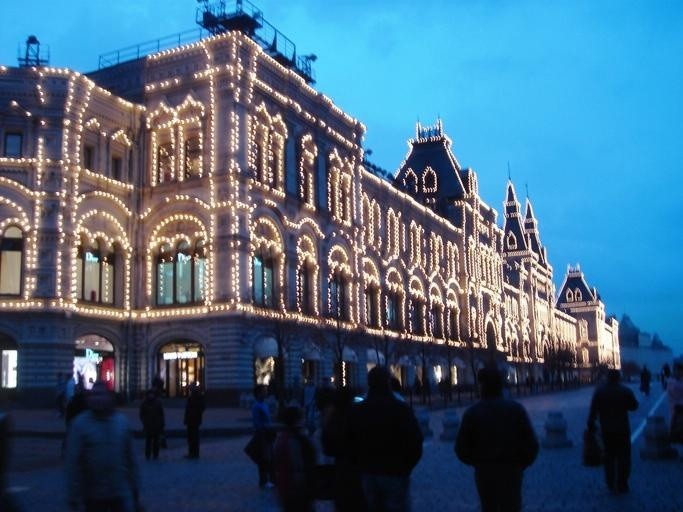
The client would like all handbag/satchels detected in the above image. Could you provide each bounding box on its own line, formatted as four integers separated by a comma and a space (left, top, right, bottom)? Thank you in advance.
582, 431, 602, 468
244, 435, 268, 465
314, 463, 336, 502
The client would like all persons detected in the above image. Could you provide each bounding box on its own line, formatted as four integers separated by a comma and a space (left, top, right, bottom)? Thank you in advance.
182, 379, 206, 459
140, 391, 165, 462
640, 358, 682, 443
588, 370, 637, 494
53, 371, 135, 511
251, 365, 425, 511
454, 369, 539, 511
